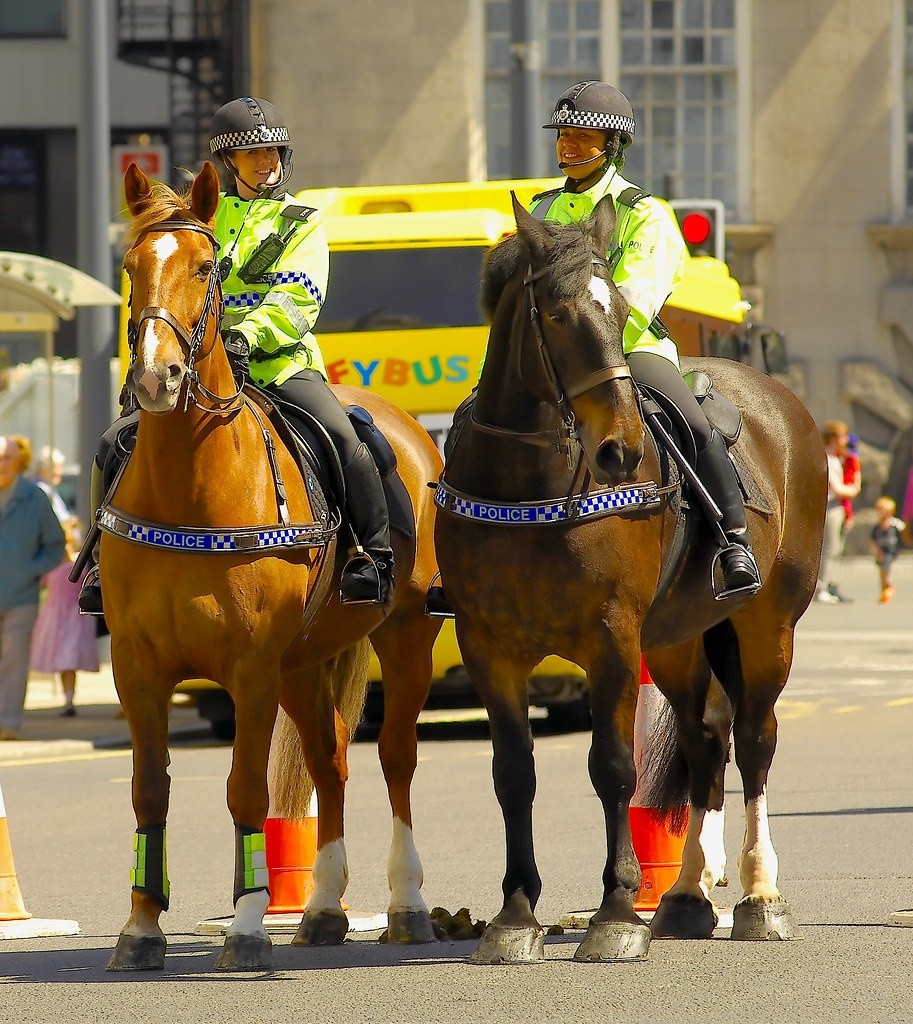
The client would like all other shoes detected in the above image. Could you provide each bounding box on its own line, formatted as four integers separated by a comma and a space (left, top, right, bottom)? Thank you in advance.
60, 704, 76, 718
815, 592, 839, 604
881, 588, 896, 604
842, 521, 854, 534
0, 727, 18, 739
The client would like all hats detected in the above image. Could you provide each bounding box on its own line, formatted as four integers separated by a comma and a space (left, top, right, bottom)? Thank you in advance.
848, 434, 859, 455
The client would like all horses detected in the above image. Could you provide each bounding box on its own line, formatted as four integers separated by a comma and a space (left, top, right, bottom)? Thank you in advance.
88, 163, 831, 974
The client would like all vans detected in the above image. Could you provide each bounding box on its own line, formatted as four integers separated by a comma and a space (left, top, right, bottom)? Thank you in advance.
167, 177, 747, 742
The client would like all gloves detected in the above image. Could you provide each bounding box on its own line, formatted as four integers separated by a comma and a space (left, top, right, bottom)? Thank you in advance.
224, 327, 251, 383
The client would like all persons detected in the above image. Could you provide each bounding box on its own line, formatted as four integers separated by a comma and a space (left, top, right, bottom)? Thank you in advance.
814, 417, 861, 604
30, 445, 71, 520
0, 436, 67, 741
28, 517, 99, 719
426, 81, 758, 603
79, 97, 393, 613
871, 496, 913, 603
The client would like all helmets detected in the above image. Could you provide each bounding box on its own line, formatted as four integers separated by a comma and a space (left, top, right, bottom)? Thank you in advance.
542, 79, 635, 135
211, 97, 293, 157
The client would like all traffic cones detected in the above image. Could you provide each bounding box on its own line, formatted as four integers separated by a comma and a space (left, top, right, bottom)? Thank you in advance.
0, 784, 80, 938
558, 657, 737, 928
196, 708, 389, 933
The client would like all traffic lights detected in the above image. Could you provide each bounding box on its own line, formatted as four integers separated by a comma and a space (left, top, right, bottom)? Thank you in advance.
666, 196, 726, 271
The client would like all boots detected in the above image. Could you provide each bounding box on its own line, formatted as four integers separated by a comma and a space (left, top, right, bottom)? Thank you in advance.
426, 585, 452, 614
695, 428, 758, 604
334, 440, 395, 608
77, 453, 106, 612
